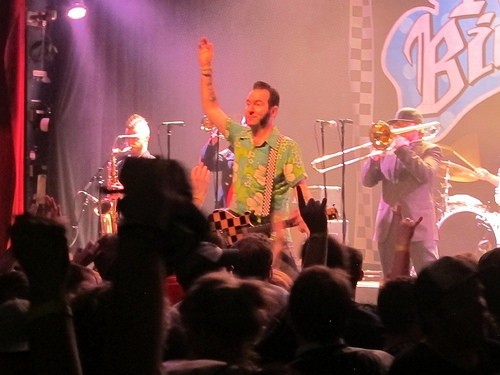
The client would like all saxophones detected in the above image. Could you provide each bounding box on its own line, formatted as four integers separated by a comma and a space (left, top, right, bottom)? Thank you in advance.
93, 147, 132, 236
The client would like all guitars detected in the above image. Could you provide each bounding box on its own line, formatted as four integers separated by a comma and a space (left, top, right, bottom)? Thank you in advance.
209, 205, 340, 250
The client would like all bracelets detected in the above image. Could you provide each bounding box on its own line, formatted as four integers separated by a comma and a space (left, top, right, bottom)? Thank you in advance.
200, 69, 213, 77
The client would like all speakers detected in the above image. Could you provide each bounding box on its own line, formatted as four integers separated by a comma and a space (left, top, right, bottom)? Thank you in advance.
289, 185, 349, 259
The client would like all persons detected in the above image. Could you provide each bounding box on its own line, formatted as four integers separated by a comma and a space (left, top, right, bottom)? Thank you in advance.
362, 107, 442, 281
0, 185, 500, 375
112, 113, 157, 235
197, 37, 310, 266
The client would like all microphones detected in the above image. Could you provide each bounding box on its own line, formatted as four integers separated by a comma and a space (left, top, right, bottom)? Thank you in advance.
161, 121, 185, 126
316, 120, 337, 128
83, 192, 103, 206
117, 133, 141, 139
339, 119, 354, 125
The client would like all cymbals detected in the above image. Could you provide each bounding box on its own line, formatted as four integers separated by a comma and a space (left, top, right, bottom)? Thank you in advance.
435, 160, 481, 182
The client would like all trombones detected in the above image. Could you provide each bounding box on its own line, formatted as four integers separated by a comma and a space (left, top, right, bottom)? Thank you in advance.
310, 120, 444, 173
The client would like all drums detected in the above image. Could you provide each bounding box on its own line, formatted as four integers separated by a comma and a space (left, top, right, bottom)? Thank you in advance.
437, 207, 500, 262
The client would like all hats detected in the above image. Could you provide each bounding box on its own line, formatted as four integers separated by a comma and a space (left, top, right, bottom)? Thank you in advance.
231, 230, 274, 276
386, 107, 423, 126
